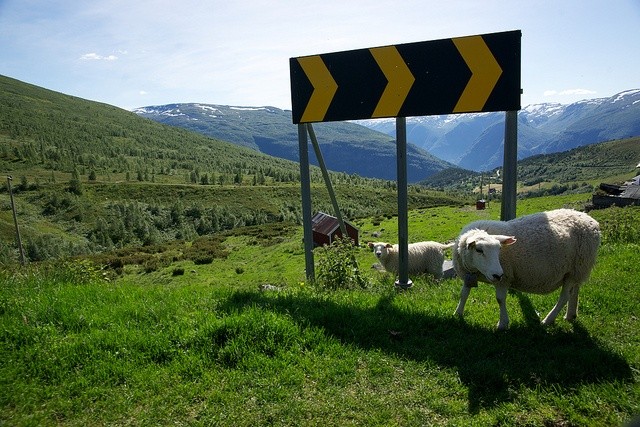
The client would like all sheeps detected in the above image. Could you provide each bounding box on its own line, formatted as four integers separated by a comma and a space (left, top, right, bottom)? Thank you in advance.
368, 242, 456, 285
453, 209, 603, 334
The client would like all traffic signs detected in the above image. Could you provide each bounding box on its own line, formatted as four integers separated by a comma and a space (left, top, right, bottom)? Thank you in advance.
288, 29, 524, 122
